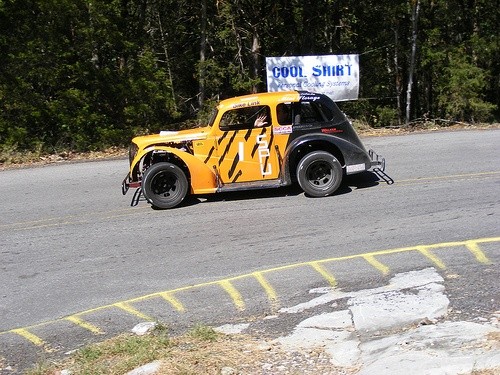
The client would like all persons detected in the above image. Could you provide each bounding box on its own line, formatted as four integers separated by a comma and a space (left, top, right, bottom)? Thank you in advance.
254, 102, 290, 128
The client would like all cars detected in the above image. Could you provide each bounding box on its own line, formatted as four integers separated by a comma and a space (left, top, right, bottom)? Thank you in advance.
119, 90, 386, 212
